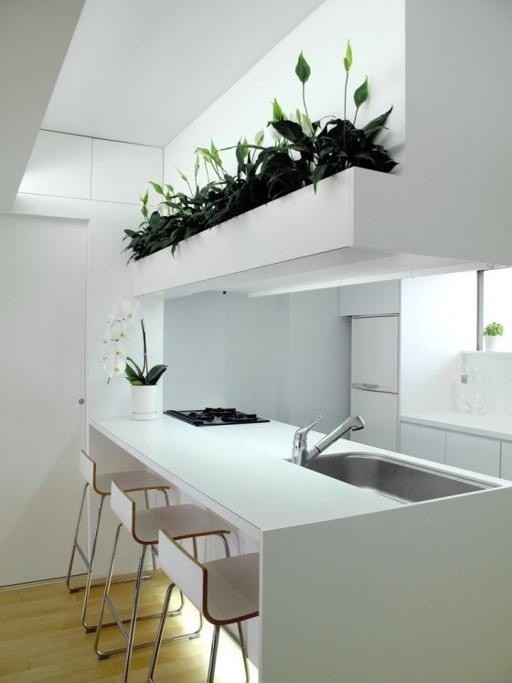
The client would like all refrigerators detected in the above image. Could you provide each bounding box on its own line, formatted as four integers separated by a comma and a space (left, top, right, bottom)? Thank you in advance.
349, 313, 398, 453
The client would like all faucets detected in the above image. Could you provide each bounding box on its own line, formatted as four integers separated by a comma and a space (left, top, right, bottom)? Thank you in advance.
293, 413, 366, 466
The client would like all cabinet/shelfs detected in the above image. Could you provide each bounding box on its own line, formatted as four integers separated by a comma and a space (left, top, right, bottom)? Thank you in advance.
396, 419, 512, 481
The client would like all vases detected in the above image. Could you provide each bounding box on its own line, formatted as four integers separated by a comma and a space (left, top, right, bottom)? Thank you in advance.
130, 385, 162, 421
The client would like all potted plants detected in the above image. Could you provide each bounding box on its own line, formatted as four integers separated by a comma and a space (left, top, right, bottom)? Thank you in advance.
482, 322, 503, 350
119, 33, 398, 270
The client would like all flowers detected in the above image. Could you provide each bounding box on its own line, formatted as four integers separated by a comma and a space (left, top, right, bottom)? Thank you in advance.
98, 304, 168, 386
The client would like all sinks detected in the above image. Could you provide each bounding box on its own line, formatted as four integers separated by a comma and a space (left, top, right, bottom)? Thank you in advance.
283, 451, 502, 506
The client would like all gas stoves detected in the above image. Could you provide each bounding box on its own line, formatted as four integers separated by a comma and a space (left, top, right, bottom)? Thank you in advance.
161, 407, 270, 427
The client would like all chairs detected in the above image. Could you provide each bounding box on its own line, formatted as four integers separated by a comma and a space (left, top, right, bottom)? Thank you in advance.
147, 529, 261, 683
64, 446, 171, 638
92, 480, 249, 683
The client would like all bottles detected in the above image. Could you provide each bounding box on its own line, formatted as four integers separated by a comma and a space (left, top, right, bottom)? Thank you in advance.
456, 373, 472, 414
